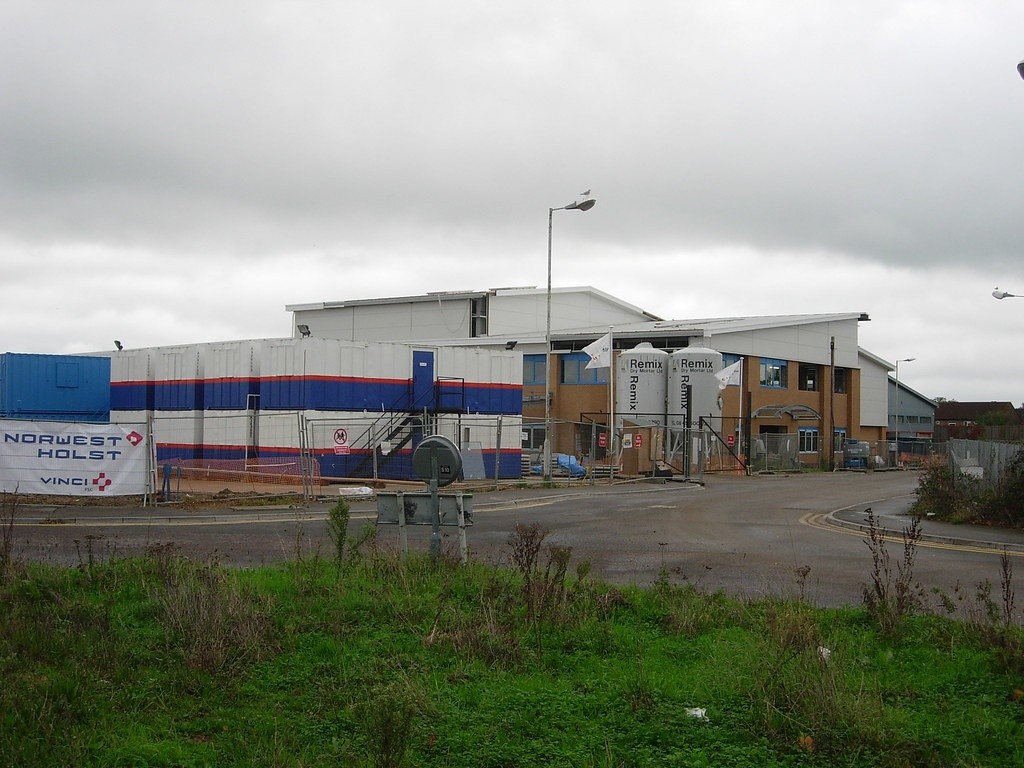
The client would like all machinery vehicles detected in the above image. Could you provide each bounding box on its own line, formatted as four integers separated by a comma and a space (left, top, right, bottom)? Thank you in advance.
843, 438, 885, 468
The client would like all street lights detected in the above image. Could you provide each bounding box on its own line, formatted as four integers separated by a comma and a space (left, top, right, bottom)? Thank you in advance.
895, 358, 917, 469
541, 190, 597, 488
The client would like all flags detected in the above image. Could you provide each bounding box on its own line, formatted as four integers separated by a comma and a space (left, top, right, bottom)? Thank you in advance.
582, 334, 610, 369
714, 361, 740, 391
